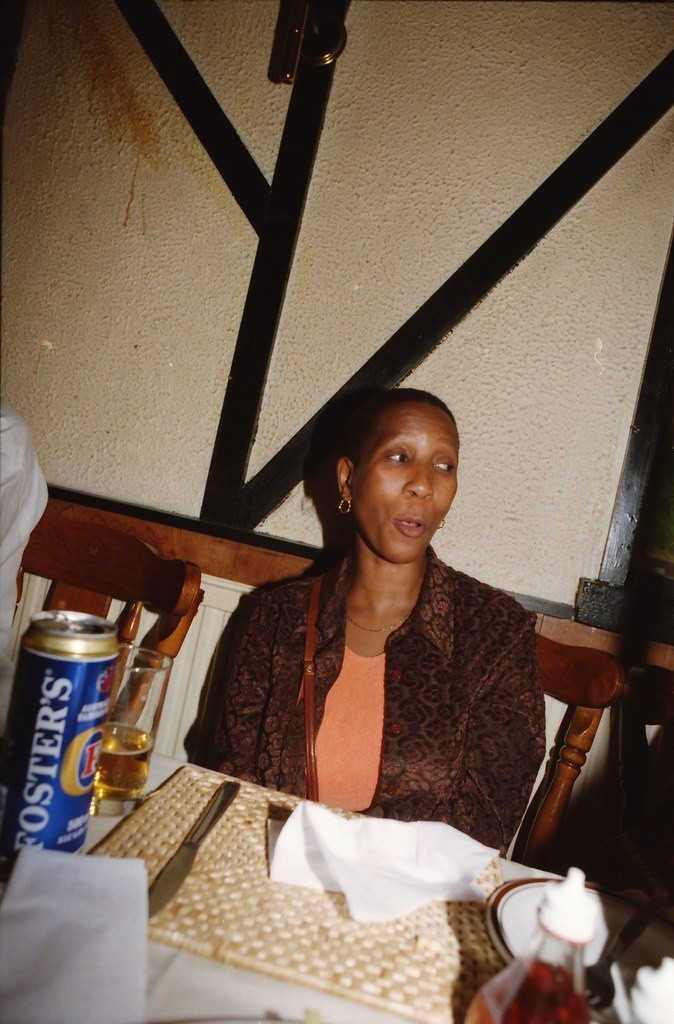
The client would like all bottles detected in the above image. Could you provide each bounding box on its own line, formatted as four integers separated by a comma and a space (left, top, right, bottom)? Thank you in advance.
461, 866, 601, 1024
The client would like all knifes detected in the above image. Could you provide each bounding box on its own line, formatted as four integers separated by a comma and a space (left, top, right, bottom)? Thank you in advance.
146, 780, 241, 919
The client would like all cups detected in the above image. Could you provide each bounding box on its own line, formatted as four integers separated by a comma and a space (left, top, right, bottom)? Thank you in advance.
88, 645, 174, 817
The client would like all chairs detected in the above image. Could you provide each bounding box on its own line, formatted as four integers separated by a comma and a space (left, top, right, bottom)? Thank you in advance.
508, 634, 621, 869
11, 512, 202, 727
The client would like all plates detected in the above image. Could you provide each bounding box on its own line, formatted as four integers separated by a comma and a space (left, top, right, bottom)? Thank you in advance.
484, 878, 674, 1024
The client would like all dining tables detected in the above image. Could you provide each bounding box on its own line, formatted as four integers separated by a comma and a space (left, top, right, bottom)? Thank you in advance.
0, 746, 673, 1024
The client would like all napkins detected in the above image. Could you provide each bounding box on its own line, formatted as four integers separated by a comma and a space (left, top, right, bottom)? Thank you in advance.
269, 798, 499, 927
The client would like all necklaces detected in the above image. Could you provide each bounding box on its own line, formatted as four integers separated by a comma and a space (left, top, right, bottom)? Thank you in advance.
347, 613, 410, 632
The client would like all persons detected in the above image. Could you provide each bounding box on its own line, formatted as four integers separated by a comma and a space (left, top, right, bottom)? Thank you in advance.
205, 387, 546, 858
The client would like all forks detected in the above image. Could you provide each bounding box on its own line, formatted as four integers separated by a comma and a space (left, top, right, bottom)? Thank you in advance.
583, 887, 671, 1012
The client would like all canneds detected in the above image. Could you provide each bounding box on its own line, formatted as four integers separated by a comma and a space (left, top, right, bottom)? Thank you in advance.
0, 608, 119, 883
